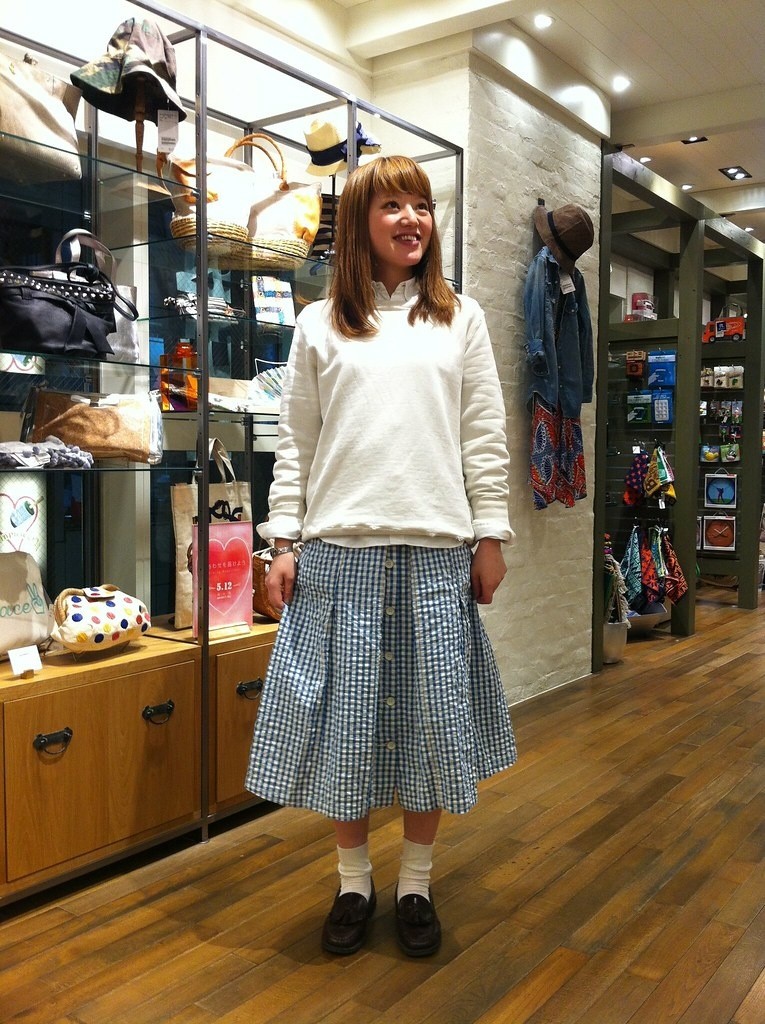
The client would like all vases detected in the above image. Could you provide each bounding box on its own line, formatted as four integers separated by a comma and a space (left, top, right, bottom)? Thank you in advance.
603, 617, 628, 665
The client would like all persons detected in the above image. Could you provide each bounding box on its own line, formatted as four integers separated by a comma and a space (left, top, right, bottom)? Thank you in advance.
244, 156, 519, 958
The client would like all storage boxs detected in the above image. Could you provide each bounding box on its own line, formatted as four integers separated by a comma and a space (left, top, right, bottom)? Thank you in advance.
696, 473, 738, 552
626, 601, 667, 639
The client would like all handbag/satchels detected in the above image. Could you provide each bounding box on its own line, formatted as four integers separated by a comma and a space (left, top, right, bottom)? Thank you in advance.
163, 294, 246, 325
19, 385, 163, 465
0, 52, 84, 185
55, 228, 142, 365
0, 551, 53, 661
0, 262, 139, 360
51, 583, 152, 650
170, 437, 255, 628
251, 275, 296, 330
171, 134, 323, 271
604, 577, 628, 663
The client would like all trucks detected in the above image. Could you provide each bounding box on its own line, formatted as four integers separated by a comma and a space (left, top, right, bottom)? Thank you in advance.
702, 316, 746, 344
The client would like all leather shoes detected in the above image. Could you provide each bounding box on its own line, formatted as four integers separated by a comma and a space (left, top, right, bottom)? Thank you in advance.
320, 874, 378, 955
393, 881, 442, 957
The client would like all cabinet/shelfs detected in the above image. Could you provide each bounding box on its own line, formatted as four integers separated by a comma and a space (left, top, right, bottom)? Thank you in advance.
0, 0, 463, 926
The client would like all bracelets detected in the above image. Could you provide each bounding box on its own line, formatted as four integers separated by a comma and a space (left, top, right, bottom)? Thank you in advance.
270, 546, 293, 559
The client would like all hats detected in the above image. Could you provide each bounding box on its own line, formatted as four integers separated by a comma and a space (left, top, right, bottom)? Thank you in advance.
70, 17, 187, 127
305, 116, 382, 176
533, 204, 594, 274
